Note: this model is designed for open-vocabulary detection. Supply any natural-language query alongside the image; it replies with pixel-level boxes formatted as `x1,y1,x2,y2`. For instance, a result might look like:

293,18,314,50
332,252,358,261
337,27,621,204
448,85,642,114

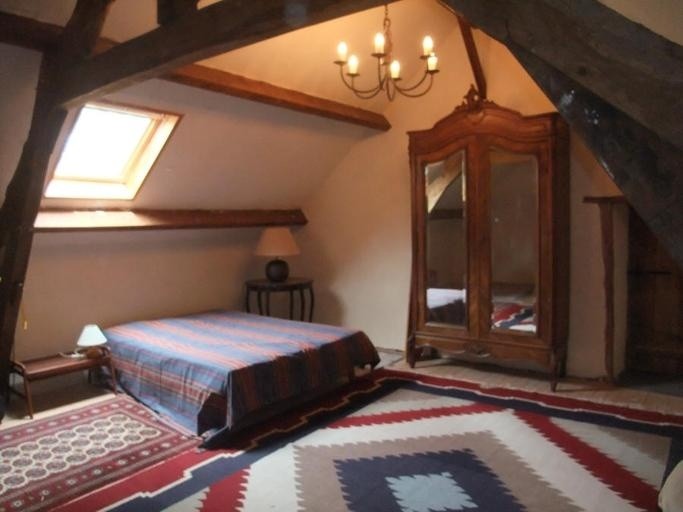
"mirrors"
488,146,539,336
421,148,470,329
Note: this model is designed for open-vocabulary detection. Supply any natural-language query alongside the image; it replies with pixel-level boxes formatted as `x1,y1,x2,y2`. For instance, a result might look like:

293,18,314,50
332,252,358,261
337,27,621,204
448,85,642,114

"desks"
243,276,315,323
3,347,120,418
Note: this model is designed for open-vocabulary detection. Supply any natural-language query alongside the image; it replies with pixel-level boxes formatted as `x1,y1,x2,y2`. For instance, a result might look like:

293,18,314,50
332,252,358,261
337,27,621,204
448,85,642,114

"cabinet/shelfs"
405,85,570,392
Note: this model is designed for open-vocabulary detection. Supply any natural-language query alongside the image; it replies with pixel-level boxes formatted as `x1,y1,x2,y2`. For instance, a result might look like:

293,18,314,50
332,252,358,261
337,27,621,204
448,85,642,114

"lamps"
254,227,302,283
333,4,441,102
76,324,105,357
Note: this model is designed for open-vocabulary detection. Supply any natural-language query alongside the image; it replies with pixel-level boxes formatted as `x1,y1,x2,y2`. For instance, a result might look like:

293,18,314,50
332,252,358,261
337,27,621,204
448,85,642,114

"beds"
102,308,367,424
